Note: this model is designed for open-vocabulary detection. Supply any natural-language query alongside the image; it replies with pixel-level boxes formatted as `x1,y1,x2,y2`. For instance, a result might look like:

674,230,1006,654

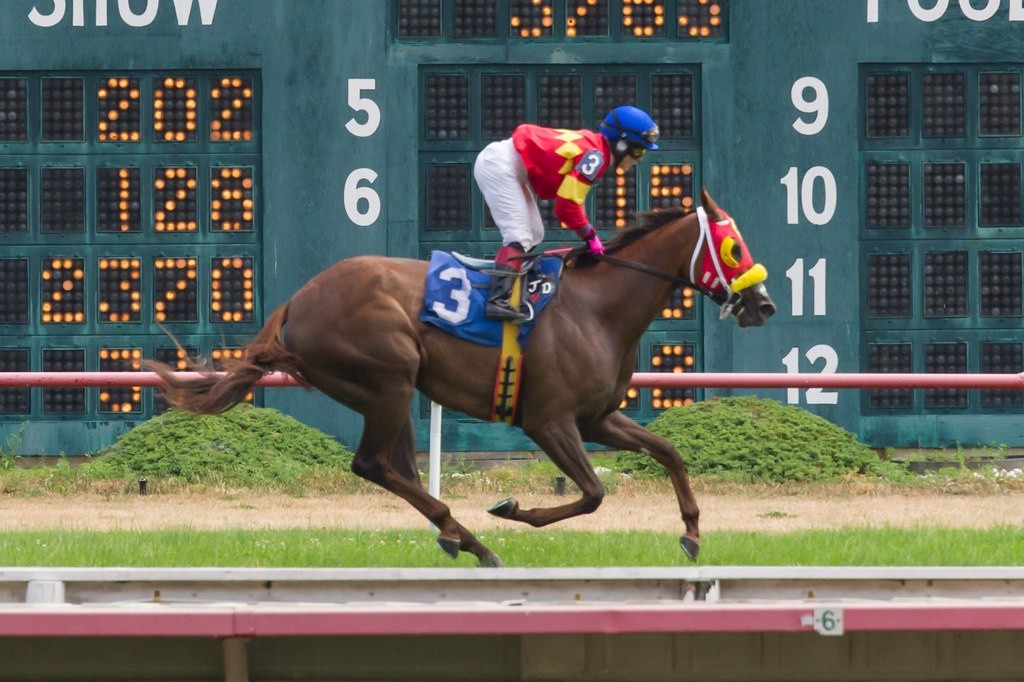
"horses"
141,185,777,568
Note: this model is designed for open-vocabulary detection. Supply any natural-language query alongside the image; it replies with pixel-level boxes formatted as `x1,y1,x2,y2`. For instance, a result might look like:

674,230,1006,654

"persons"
473,105,660,324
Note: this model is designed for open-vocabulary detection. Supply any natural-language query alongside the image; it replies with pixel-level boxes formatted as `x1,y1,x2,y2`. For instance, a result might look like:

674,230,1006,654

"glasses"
642,126,659,143
627,143,647,159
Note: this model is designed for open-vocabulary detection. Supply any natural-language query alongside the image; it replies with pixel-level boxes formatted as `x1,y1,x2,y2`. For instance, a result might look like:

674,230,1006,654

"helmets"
599,106,659,151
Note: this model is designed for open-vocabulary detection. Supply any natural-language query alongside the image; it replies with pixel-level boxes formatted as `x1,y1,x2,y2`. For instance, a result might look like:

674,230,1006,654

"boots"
483,241,536,322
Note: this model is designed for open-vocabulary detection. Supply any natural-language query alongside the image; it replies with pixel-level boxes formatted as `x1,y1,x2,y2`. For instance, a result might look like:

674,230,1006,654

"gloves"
585,228,605,256
